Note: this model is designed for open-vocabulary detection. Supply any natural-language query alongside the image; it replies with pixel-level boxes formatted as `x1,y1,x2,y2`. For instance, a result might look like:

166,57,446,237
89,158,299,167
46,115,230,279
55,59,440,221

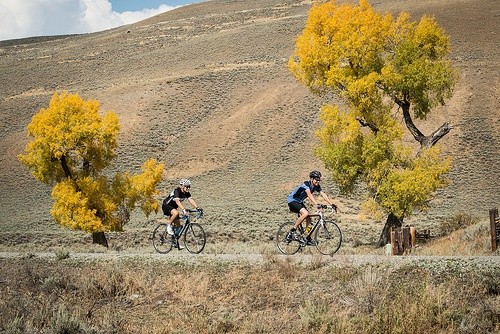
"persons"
285,170,337,246
162,179,203,249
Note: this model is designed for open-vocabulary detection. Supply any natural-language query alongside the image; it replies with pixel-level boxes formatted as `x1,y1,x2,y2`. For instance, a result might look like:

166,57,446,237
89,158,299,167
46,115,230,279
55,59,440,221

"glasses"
315,178,320,181
184,185,190,189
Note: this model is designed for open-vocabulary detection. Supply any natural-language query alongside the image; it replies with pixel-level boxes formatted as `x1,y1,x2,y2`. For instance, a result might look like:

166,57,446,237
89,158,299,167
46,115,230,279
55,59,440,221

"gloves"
331,204,337,210
317,204,324,210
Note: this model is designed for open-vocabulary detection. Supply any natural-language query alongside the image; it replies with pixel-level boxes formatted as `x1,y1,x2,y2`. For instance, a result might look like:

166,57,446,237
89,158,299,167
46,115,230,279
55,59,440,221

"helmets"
180,178,191,186
309,170,322,178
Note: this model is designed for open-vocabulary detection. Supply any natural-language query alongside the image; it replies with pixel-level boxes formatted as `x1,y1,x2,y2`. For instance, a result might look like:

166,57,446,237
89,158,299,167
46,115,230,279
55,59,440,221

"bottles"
305,223,313,234
176,226,183,235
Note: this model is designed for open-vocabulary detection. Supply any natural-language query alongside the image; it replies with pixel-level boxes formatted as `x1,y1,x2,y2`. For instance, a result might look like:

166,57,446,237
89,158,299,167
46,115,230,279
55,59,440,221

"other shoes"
179,245,186,249
290,229,299,241
166,224,175,236
309,238,319,245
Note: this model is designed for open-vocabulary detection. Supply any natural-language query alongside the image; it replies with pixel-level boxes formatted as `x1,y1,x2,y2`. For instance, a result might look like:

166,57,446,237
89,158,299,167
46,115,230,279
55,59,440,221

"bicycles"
276,204,342,255
153,207,206,254
